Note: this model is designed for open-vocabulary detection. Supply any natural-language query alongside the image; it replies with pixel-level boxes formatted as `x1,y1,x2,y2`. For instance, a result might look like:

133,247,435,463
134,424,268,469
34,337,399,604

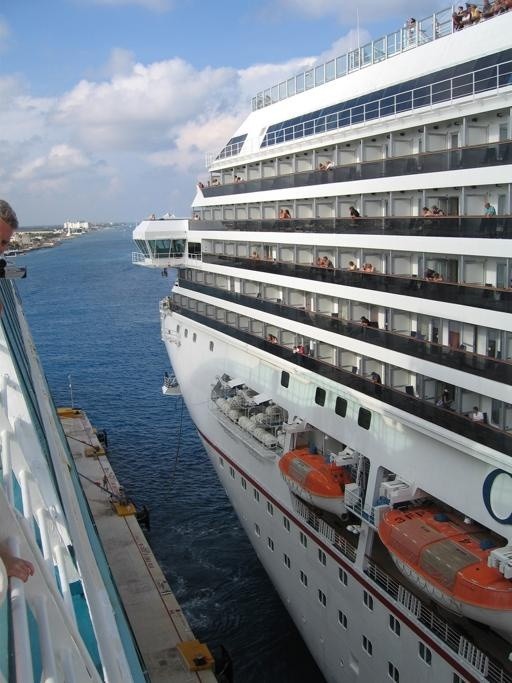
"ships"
0,236,227,681
129,0,510,683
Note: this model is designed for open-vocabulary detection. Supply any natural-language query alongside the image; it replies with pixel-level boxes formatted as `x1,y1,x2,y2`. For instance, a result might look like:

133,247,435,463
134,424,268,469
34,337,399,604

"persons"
471,405,483,422
371,370,382,394
234,174,239,182
237,175,244,181
1,199,18,257
279,208,284,217
0,538,35,581
293,345,301,354
194,214,201,220
433,206,450,232
404,16,418,47
324,156,335,181
272,335,279,344
267,333,274,342
441,388,453,407
359,315,370,327
214,178,220,185
451,0,512,31
103,474,109,488
253,250,259,259
283,209,291,218
315,160,326,183
424,271,442,281
199,181,204,187
313,253,375,272
348,204,361,226
297,345,305,355
418,205,433,226
483,201,499,236
